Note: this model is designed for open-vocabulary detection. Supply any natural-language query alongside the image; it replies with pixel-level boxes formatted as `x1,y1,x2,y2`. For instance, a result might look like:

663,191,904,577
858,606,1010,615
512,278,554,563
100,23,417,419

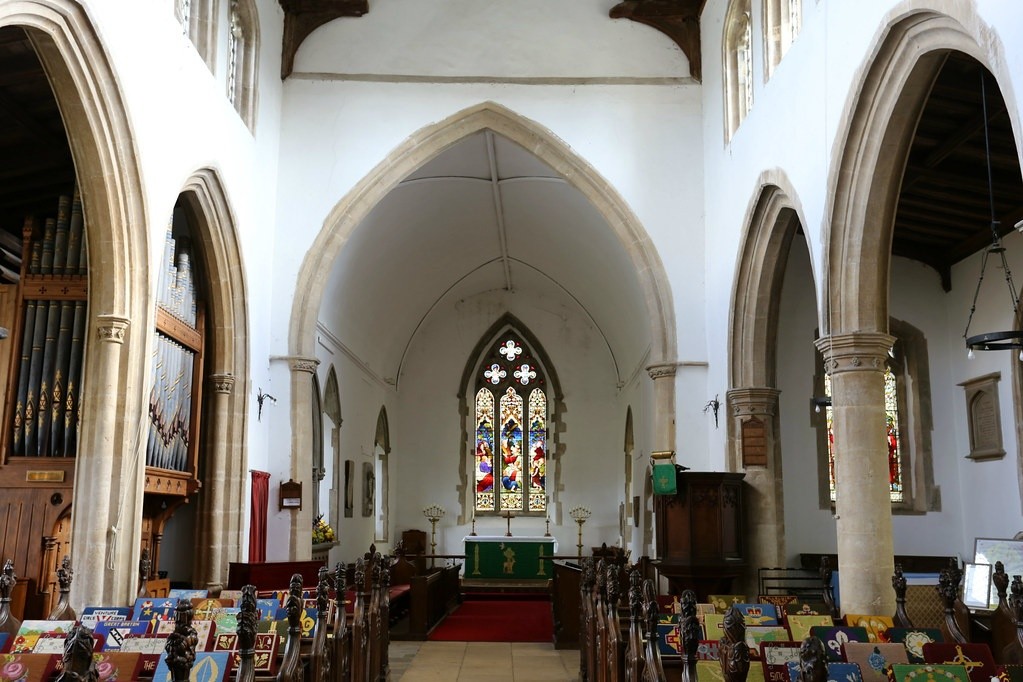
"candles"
568,504,592,514
547,504,550,521
423,504,445,513
473,505,474,519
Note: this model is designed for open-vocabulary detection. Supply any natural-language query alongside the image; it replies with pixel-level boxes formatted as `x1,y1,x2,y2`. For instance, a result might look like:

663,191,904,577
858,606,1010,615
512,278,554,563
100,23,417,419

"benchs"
578,557,1023,682
0,552,390,682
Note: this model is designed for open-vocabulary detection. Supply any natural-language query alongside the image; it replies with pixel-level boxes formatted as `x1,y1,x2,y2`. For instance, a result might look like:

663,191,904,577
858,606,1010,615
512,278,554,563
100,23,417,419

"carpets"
427,600,554,641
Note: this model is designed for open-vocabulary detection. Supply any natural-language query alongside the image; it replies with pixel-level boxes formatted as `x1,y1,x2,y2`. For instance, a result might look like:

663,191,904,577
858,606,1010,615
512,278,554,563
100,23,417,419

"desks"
464,536,555,580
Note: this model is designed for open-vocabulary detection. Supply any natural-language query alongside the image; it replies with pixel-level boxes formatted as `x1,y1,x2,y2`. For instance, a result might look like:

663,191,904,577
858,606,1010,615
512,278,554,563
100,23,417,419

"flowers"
312,514,334,545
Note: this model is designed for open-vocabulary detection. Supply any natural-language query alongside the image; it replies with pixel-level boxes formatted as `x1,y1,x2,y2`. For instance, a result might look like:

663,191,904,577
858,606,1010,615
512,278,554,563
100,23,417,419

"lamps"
961,64,1023,362
